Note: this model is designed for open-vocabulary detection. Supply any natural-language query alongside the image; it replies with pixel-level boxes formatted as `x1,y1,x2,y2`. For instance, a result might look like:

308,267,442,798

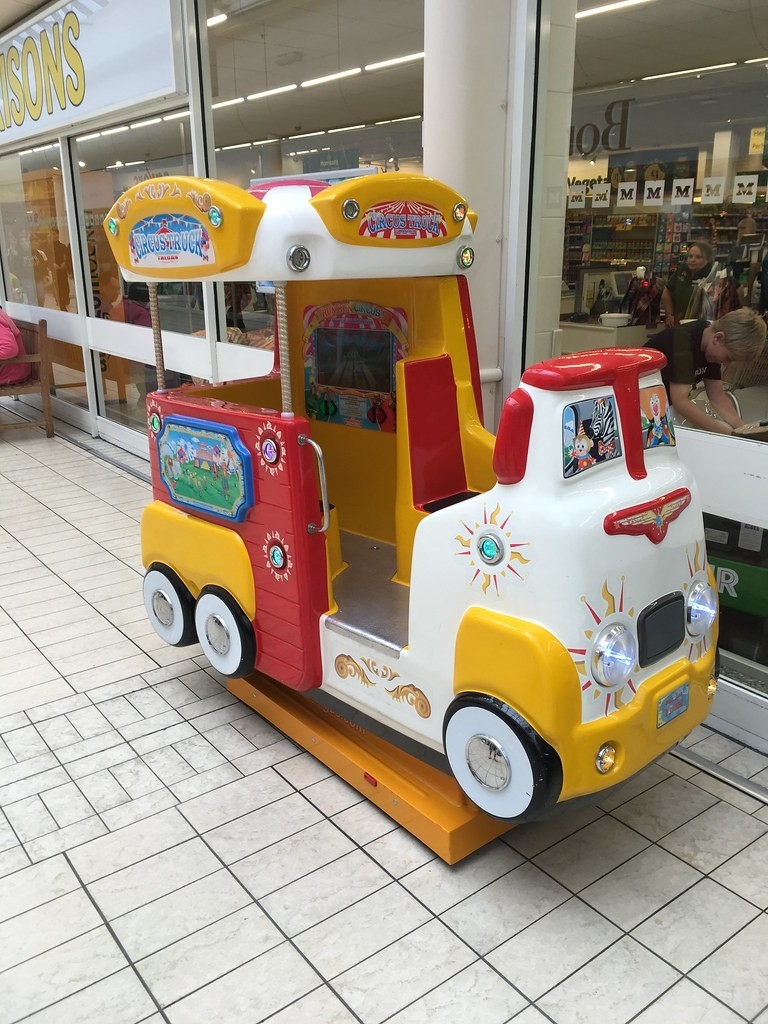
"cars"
98,163,727,823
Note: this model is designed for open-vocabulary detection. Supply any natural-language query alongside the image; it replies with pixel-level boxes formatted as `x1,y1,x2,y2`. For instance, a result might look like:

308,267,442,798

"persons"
10,236,47,307
663,239,716,330
98,274,121,318
110,283,158,408
0,307,31,385
38,229,74,312
642,308,767,436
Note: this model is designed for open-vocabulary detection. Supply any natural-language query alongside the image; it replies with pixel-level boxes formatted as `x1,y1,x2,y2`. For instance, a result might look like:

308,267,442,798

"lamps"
17,1,767,171
207,14,227,27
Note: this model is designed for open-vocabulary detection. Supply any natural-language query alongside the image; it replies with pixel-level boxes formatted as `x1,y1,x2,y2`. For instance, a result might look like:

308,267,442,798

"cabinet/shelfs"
590,214,659,270
687,212,768,268
565,219,584,286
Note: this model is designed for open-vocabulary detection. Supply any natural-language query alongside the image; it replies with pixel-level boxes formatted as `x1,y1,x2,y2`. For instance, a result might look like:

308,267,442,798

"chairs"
393,352,485,585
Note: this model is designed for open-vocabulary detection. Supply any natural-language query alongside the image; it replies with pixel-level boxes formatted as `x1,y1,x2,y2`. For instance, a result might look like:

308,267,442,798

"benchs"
0,317,53,439
